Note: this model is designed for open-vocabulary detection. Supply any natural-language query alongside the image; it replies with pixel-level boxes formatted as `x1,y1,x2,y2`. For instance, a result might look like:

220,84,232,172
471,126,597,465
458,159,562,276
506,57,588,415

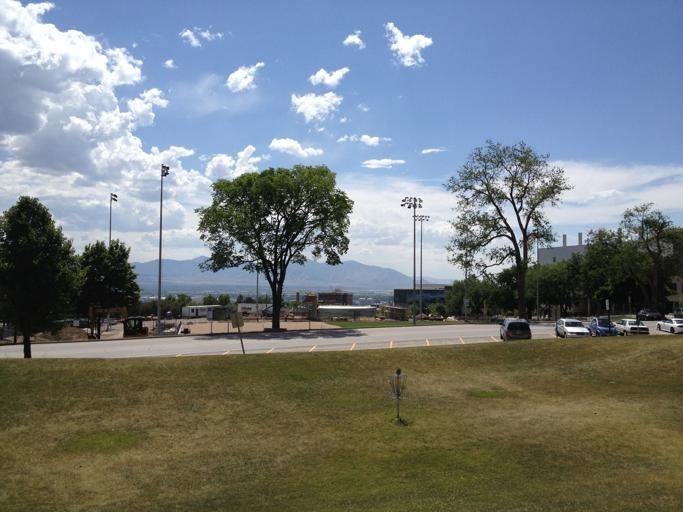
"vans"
498,318,532,342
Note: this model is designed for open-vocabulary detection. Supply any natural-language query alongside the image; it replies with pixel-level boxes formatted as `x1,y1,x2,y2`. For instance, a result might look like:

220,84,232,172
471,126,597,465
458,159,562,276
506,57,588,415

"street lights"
157,164,170,322
400,196,430,325
605,299,611,336
109,193,118,249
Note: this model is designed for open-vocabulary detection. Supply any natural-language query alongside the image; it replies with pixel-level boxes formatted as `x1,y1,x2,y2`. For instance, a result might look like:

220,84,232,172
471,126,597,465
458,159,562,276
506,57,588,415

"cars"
555,308,683,339
491,315,505,321
